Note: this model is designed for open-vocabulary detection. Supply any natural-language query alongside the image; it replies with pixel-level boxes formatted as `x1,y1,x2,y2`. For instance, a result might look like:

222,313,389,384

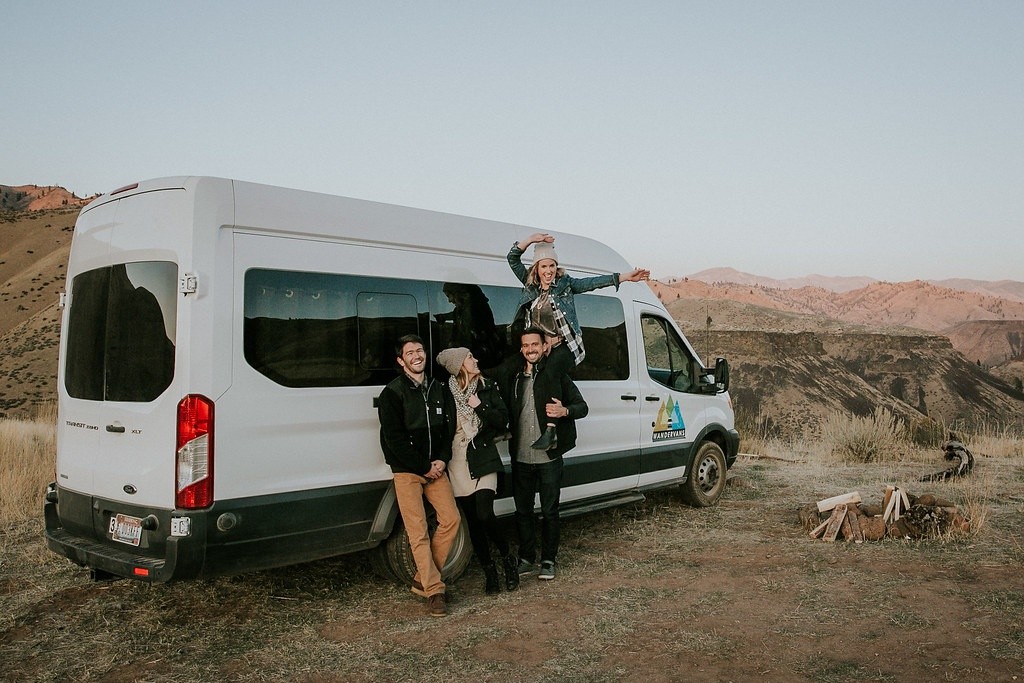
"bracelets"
566,408,568,415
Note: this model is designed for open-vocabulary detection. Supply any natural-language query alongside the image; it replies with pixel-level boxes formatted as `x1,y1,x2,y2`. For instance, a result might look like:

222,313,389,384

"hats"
436,347,470,377
531,242,558,267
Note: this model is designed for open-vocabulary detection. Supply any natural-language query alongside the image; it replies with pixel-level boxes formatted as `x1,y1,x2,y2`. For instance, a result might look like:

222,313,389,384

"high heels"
493,430,512,443
532,426,557,451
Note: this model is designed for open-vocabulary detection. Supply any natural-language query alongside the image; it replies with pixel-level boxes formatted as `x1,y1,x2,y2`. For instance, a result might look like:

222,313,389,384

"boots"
502,553,520,592
483,560,499,596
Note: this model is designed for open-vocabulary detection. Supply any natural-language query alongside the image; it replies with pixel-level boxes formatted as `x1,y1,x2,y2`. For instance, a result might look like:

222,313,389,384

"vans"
42,174,741,593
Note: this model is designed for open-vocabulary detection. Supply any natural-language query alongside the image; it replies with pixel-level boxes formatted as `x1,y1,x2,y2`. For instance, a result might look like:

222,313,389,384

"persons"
504,233,650,580
378,334,461,616
436,346,521,595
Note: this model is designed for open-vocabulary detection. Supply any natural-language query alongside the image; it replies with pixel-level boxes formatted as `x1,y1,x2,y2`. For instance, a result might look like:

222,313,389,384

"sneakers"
428,594,446,617
538,561,555,579
504,559,534,577
410,580,429,599
